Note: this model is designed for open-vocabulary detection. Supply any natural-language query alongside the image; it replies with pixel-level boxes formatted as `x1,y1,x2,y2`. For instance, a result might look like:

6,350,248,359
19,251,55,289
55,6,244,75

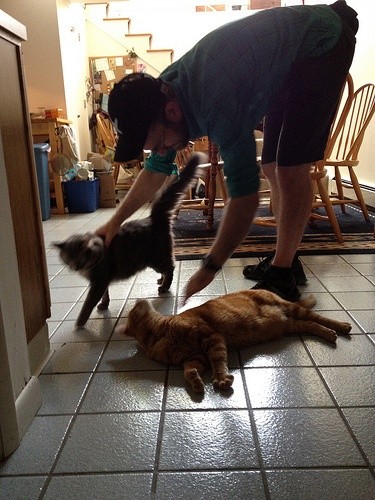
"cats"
51,152,209,329
114,290,353,393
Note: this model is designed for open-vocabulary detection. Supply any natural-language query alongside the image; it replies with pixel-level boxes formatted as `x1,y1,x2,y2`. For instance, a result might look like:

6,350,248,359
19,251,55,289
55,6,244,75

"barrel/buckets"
33,143,50,221
62,177,98,213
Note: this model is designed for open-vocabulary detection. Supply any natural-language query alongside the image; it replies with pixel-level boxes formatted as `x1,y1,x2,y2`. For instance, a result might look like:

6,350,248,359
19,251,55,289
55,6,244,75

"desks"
31,117,73,215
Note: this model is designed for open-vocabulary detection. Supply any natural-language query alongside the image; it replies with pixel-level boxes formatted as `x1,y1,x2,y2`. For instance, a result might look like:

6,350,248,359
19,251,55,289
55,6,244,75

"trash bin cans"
33,143,51,221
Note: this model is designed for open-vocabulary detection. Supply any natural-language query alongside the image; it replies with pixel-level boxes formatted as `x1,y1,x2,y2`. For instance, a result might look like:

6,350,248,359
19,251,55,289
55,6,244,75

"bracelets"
200,253,222,273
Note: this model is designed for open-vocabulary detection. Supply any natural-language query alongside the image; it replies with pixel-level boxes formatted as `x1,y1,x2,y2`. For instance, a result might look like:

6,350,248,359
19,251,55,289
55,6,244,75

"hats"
108,72,159,162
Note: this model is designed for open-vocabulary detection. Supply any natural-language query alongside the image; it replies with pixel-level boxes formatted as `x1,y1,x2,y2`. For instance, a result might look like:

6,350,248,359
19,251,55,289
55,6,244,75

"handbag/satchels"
329,0,359,35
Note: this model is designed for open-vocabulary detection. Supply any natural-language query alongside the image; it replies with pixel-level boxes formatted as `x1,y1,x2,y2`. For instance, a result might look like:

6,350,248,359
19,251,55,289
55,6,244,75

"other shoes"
249,274,302,302
243,251,308,284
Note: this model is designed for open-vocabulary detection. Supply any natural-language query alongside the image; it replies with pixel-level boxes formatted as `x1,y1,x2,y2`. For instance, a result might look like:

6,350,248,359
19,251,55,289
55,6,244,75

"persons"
95,0,359,308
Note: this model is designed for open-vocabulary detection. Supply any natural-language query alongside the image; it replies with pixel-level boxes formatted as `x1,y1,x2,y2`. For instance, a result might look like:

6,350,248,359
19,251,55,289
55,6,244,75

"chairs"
173,141,229,220
300,71,345,243
310,82,375,222
96,114,143,194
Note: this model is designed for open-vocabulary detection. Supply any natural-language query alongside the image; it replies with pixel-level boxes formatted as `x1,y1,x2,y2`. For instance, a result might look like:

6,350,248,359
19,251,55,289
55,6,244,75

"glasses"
151,126,165,155
